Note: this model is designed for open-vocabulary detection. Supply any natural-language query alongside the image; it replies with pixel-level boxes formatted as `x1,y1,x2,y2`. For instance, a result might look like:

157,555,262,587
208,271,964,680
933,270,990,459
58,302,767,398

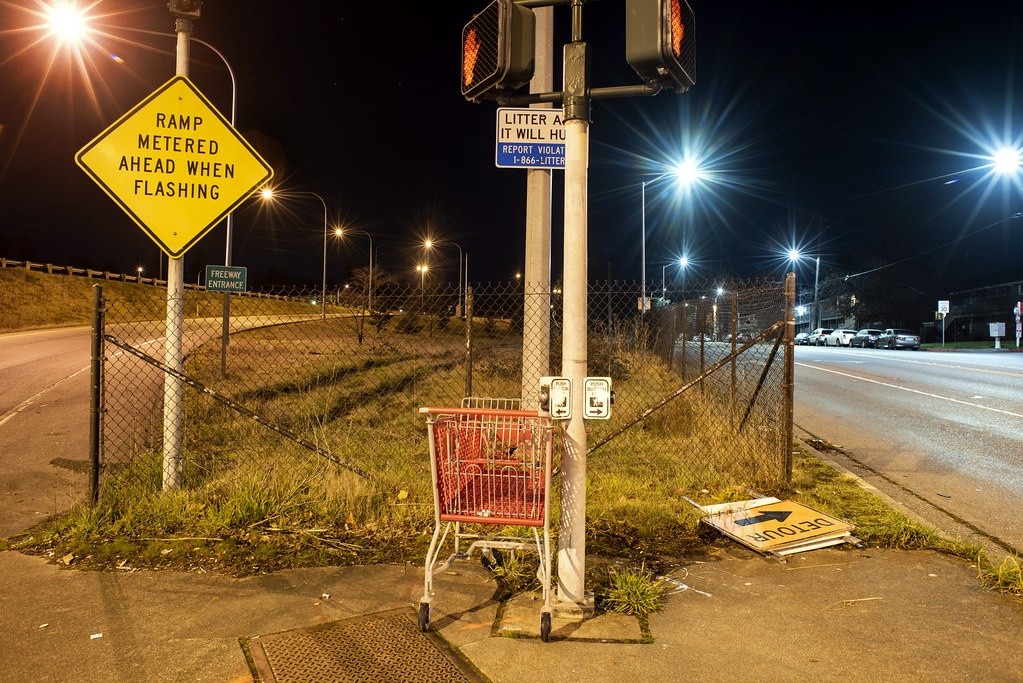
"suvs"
807,328,834,346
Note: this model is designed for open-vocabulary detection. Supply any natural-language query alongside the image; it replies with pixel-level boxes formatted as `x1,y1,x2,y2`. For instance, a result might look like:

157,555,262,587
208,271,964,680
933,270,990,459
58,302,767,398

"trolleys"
417,396,556,642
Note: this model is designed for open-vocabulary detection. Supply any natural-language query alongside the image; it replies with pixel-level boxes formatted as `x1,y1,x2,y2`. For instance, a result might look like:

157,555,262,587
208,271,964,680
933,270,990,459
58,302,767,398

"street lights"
663,258,688,304
642,165,694,313
426,241,462,314
338,228,372,313
58,8,237,372
418,264,429,312
267,190,325,320
792,252,819,330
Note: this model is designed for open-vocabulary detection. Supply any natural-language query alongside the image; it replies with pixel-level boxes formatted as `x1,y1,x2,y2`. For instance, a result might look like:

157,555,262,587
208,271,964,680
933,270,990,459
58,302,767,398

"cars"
795,332,809,345
736,333,753,343
849,329,882,349
824,329,858,347
874,329,921,350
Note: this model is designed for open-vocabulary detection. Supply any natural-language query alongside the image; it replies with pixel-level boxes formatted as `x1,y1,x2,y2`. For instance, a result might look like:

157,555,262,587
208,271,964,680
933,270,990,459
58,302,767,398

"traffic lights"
625,0,697,96
461,0,535,99
